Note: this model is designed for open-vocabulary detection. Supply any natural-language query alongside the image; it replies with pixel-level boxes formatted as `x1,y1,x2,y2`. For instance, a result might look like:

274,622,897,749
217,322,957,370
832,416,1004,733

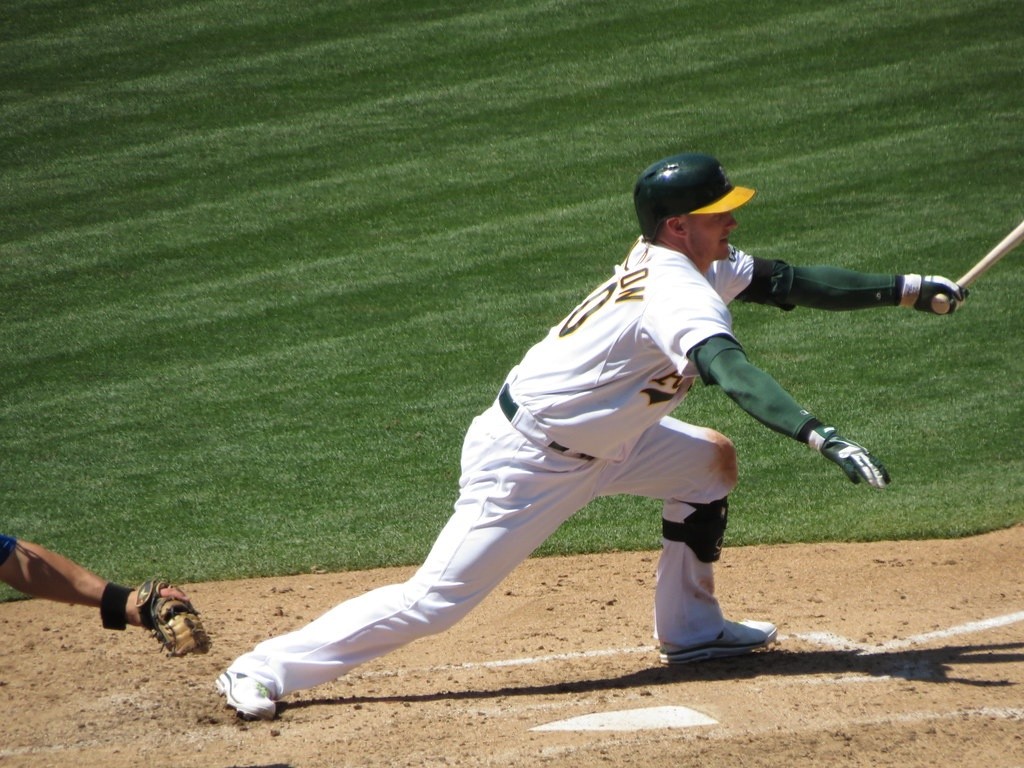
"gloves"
899,271,971,315
809,425,891,489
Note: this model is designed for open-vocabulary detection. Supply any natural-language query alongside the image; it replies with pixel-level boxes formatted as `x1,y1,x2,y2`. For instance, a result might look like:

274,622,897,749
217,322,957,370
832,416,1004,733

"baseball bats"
932,220,1024,314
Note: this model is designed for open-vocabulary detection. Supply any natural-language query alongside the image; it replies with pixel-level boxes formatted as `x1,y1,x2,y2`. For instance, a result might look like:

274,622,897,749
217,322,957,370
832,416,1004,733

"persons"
214,154,970,720
0,535,213,658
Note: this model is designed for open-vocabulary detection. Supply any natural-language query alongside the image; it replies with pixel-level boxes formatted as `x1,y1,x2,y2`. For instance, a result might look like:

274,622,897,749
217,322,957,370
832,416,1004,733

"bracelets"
99,581,134,633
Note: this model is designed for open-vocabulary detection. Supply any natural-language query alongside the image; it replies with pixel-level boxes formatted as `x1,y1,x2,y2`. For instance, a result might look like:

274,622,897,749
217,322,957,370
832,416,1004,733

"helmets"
634,154,756,244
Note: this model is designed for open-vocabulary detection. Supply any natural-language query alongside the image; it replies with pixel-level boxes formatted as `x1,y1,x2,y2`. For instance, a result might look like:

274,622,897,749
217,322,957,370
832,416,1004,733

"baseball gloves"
137,577,210,659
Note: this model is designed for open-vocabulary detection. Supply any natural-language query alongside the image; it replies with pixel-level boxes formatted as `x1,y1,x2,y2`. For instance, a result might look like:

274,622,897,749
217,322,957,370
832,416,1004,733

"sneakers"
659,621,777,664
216,656,277,719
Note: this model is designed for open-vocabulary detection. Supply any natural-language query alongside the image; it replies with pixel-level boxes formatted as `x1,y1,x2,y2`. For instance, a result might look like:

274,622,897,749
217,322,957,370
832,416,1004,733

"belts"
499,387,596,460
